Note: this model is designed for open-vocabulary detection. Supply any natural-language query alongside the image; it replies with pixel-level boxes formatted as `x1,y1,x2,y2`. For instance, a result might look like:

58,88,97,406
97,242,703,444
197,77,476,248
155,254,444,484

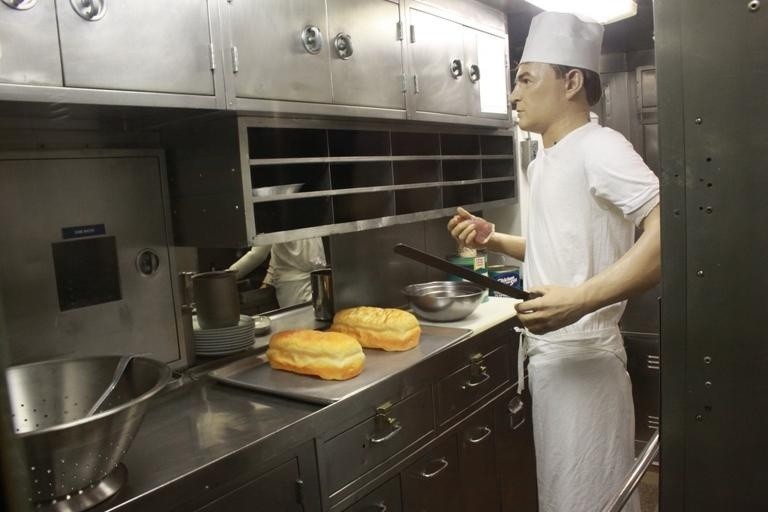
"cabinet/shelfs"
227,0,409,122
412,1,519,128
0,0,225,107
165,115,521,248
218,315,537,511
168,381,322,510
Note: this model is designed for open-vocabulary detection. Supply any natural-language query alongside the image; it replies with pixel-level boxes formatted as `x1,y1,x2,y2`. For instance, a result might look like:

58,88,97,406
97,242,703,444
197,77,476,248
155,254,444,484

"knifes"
393,242,540,301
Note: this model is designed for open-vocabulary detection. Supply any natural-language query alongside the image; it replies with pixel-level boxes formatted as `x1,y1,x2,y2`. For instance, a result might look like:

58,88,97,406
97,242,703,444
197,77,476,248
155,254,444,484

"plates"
250,182,303,197
190,315,256,356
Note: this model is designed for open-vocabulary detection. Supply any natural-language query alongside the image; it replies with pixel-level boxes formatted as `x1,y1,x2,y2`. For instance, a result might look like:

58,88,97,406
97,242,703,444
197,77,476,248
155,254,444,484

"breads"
266,329,366,380
330,306,421,352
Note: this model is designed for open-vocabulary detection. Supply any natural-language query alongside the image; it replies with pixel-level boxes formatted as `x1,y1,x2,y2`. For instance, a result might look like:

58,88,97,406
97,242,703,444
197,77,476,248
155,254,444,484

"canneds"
488,265,521,298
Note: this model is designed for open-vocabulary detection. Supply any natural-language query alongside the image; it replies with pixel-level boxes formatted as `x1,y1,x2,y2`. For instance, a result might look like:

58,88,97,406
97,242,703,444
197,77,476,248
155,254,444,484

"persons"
446,10,662,512
224,236,327,308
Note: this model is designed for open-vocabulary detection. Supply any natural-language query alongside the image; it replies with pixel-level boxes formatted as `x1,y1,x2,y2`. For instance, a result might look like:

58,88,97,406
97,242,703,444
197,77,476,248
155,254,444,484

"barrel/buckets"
448,253,488,302
487,263,520,295
310,270,331,321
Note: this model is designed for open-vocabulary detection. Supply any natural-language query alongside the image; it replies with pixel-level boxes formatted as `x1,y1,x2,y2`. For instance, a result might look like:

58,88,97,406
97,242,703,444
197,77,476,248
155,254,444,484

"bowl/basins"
1,356,173,508
252,316,271,334
402,280,486,321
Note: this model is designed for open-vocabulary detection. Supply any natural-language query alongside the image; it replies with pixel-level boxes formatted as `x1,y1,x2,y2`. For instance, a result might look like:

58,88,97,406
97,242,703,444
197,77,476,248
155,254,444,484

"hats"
519,11,606,75
